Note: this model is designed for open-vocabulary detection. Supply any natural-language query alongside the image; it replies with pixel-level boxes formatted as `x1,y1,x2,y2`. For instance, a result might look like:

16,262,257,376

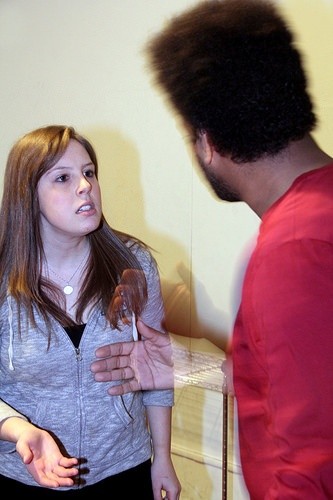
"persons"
0,125,182,500
91,1,333,500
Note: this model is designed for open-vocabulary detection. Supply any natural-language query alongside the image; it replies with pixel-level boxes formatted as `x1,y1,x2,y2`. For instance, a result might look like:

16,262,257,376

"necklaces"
49,250,89,295
